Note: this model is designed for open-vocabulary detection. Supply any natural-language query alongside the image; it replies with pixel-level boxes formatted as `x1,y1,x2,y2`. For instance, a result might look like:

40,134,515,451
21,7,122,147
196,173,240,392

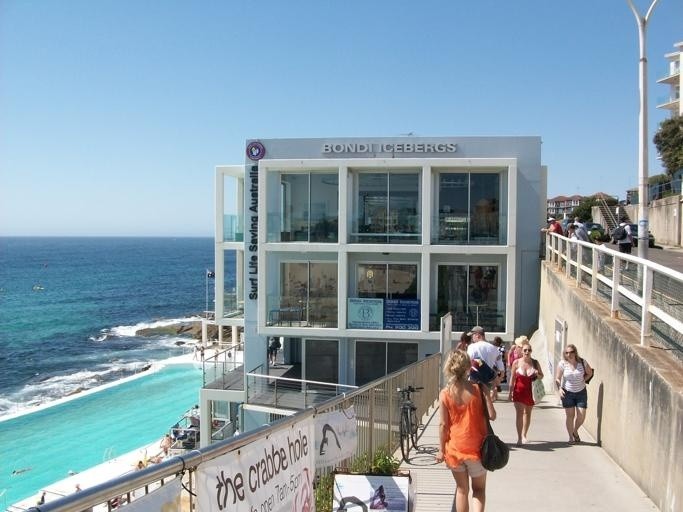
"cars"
610,223,655,248
562,217,605,239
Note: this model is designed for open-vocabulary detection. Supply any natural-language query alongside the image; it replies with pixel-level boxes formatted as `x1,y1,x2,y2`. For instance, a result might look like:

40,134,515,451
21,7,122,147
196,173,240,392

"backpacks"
613,224,629,240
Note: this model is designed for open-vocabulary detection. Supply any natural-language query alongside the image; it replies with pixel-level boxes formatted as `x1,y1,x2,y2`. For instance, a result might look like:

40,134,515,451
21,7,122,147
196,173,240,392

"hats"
514,336,529,348
619,216,629,222
547,218,555,223
465,326,484,336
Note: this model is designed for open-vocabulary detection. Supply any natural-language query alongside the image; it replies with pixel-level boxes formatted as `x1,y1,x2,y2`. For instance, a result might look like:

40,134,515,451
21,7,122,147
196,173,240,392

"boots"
568,432,580,444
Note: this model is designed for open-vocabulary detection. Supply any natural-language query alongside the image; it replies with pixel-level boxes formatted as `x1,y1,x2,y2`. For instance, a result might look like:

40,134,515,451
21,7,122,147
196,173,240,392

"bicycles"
397,386,424,462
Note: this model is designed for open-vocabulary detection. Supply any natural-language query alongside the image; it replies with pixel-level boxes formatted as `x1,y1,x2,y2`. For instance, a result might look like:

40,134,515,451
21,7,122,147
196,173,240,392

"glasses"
523,348,531,352
566,352,574,354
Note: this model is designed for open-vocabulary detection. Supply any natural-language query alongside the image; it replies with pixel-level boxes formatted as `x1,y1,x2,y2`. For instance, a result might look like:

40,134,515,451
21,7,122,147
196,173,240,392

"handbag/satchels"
479,433,508,472
468,359,497,384
581,357,594,384
531,378,545,404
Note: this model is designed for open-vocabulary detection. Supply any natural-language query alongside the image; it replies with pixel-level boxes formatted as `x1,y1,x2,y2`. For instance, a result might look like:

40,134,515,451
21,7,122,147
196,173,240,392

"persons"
489,337,508,393
480,276,489,300
433,348,498,512
505,335,528,364
572,217,585,230
191,345,198,360
616,216,635,271
199,346,204,360
539,217,563,235
565,222,590,242
212,348,219,364
226,350,232,361
268,337,276,365
134,460,145,471
160,433,171,454
504,339,543,447
463,325,501,394
627,200,630,205
553,343,593,445
455,332,471,353
